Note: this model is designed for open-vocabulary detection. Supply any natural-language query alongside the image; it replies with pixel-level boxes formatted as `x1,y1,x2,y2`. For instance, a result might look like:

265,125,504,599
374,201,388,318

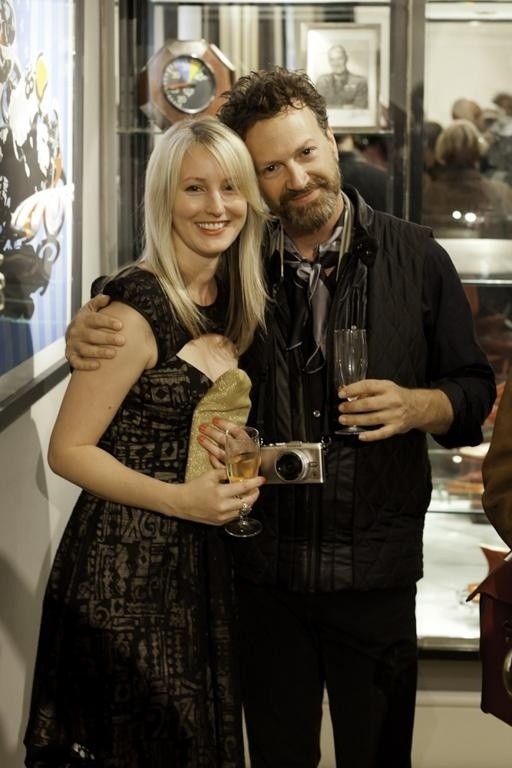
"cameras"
257,442,324,485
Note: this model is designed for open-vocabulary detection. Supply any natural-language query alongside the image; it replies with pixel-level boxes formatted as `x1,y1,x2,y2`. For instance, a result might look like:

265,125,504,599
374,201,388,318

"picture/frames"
299,22,381,135
0,0,85,434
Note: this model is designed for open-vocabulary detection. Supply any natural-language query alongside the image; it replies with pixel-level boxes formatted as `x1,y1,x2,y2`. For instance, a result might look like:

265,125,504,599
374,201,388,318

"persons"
313,44,369,109
306,87,512,325
21,112,279,768
59,64,501,767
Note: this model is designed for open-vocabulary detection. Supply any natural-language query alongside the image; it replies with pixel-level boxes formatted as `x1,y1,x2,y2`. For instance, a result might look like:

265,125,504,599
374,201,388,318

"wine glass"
332,327,372,436
222,427,264,538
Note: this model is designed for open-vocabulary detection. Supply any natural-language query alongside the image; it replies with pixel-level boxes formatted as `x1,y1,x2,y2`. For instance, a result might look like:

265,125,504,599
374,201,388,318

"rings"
239,502,252,517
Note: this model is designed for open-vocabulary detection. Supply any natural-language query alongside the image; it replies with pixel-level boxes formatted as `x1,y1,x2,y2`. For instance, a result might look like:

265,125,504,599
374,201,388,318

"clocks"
134,41,237,135
